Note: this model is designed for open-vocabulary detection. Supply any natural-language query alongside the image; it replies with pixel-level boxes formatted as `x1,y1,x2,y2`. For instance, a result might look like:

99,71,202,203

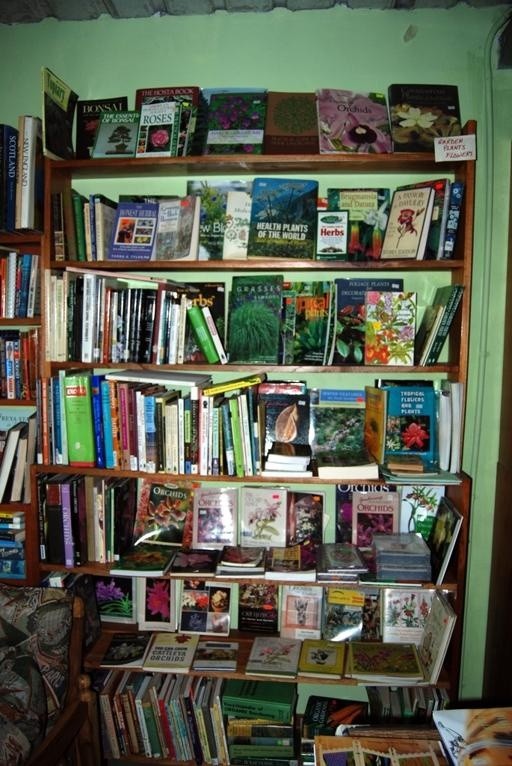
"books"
432,707,512,766
0,63,462,766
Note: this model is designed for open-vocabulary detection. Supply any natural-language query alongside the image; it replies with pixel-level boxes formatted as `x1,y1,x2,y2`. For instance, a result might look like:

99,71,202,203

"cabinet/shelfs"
0,121,478,766
